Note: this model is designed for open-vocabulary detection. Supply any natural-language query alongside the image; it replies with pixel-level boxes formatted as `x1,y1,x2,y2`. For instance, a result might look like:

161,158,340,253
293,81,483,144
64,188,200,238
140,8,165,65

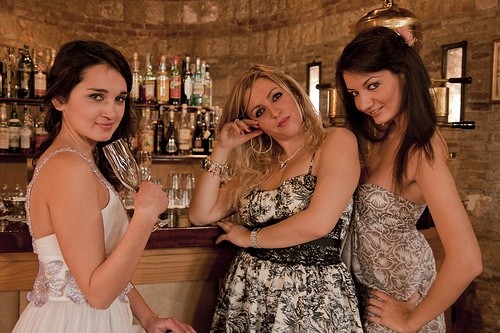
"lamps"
356,0,419,28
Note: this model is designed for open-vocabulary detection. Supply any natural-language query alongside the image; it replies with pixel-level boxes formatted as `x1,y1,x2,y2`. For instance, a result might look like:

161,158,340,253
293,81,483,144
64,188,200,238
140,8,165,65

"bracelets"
200,157,228,176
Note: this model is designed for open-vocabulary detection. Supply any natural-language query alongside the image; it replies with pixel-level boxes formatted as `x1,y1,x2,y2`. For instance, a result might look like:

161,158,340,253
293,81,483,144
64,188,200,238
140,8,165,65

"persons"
335,25,483,333
187,64,363,333
9,39,197,333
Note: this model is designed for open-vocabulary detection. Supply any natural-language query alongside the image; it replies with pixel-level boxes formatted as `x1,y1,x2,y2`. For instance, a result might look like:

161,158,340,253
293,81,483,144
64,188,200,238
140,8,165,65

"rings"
235,119,239,122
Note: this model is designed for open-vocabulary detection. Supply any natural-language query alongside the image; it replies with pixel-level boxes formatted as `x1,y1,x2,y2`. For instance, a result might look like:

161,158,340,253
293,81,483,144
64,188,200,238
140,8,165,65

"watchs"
250,228,260,249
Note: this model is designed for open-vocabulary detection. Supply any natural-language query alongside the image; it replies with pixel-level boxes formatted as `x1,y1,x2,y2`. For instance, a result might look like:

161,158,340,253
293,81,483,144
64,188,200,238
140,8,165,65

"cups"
150,173,197,228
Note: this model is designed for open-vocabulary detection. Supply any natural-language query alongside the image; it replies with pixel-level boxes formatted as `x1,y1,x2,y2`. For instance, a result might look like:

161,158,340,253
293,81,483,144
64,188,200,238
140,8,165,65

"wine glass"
102,137,171,233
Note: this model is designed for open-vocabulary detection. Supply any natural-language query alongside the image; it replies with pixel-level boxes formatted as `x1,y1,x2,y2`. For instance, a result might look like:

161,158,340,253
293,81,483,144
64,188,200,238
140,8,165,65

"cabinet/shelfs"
0,97,212,163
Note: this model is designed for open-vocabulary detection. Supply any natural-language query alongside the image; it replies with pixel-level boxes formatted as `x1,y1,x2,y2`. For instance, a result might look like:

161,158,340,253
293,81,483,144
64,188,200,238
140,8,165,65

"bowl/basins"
3,197,26,213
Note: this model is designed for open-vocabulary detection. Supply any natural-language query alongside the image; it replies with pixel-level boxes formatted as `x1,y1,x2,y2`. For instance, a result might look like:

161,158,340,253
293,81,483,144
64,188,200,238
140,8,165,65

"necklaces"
278,145,303,171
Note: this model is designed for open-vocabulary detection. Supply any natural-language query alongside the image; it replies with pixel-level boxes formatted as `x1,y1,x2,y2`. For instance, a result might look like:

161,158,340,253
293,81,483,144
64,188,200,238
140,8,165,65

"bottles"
0,44,223,156
